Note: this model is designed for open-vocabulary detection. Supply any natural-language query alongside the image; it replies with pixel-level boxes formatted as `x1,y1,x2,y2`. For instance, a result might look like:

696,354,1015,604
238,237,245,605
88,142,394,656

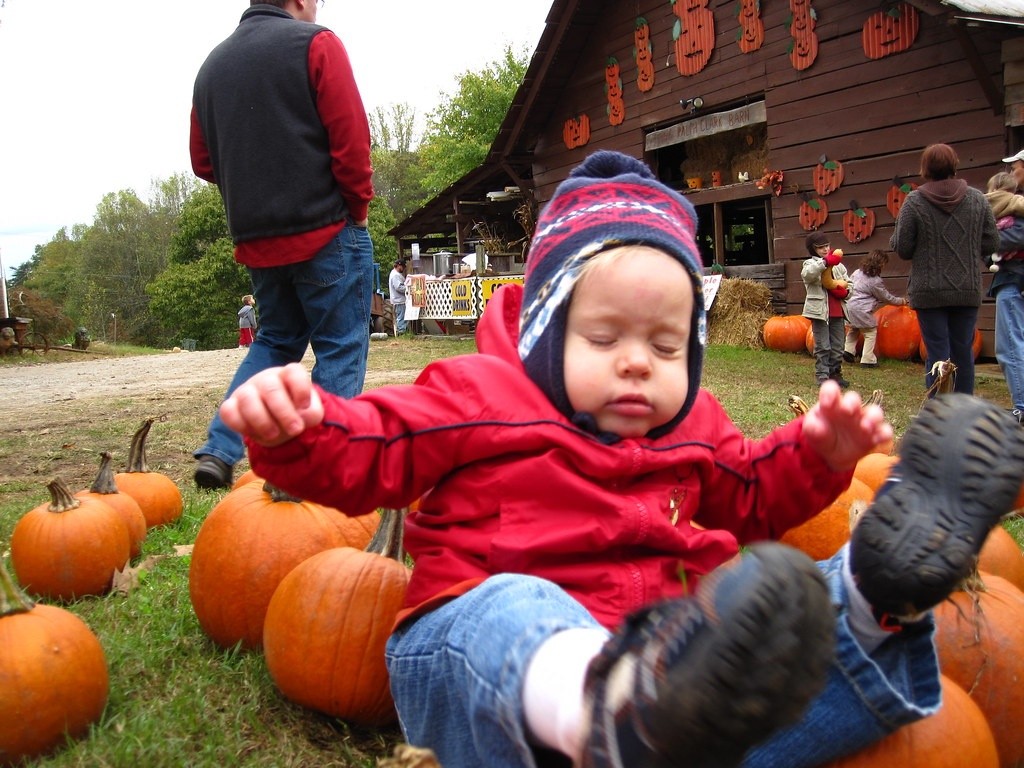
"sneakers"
1008,407,1024,424
194,452,233,493
851,391,1024,635
580,543,834,768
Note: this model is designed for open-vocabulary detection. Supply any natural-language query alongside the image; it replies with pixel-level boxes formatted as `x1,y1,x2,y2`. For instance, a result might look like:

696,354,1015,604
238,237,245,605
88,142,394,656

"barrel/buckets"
432,249,455,276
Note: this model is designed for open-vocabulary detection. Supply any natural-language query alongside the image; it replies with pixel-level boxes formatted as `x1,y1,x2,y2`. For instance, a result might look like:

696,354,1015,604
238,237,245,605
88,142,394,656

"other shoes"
863,363,880,368
843,352,854,363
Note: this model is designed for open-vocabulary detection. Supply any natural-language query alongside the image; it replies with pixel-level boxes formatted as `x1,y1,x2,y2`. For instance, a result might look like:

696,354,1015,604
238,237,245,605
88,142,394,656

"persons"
188,1,374,497
237,295,257,349
388,259,409,335
800,231,853,388
403,255,423,336
219,151,1024,768
980,149,1024,426
982,172,1024,274
889,142,1004,400
844,248,911,369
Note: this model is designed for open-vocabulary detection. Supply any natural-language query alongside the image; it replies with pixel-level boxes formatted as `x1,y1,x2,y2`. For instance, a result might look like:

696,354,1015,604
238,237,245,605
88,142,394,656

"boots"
830,369,849,387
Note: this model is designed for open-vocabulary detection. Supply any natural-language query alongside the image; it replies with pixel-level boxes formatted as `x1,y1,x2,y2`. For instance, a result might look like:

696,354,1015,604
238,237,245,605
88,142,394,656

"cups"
453,263,470,274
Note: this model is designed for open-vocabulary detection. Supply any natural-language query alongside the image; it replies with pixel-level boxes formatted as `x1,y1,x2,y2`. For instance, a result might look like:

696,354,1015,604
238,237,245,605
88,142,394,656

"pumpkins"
773,360,1024,768
822,249,849,290
764,298,982,365
0,415,415,767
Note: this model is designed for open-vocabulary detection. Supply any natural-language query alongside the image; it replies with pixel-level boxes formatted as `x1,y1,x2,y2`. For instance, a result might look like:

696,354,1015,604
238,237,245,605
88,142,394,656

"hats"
1002,150,1024,163
517,151,706,444
806,231,830,257
394,259,406,265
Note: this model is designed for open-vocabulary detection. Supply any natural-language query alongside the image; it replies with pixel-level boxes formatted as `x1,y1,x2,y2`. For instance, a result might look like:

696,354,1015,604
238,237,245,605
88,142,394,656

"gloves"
830,284,848,300
823,249,842,266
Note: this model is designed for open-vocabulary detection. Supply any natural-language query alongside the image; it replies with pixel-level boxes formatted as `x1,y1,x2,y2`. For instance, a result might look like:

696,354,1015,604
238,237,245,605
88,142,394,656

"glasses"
317,0,325,8
